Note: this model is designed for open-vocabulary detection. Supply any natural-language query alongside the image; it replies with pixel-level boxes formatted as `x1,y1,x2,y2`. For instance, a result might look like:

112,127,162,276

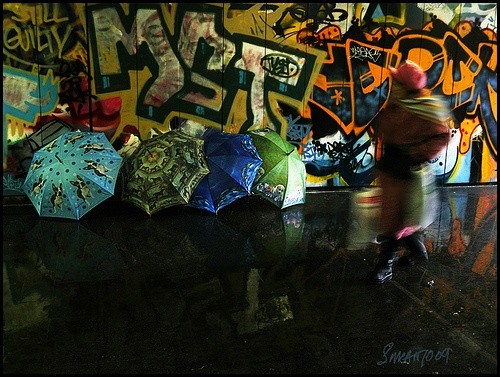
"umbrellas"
250,129,306,210
20,129,123,222
120,128,211,217
184,130,263,212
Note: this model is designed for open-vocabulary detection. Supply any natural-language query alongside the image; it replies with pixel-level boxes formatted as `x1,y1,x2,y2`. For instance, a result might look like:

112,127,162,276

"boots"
366,251,394,285
397,250,428,266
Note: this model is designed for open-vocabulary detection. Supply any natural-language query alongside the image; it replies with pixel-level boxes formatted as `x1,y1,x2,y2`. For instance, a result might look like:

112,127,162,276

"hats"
388,59,426,89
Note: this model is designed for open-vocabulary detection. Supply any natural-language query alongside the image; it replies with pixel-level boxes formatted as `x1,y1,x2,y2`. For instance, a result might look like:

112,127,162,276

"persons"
373,62,451,281
